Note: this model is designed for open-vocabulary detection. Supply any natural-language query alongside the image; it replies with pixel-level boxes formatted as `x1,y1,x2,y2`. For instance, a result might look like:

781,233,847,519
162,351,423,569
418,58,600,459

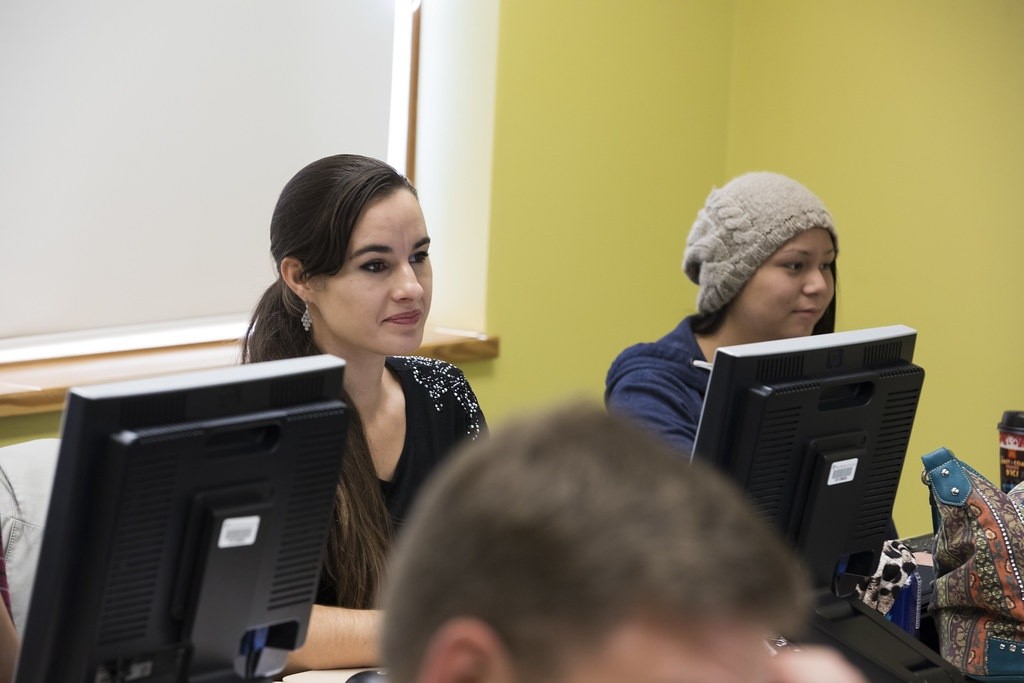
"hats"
684,171,834,314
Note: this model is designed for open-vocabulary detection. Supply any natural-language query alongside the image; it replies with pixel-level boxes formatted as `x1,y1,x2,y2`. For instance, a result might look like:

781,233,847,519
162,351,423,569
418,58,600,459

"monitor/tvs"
684,326,969,683
12,354,353,683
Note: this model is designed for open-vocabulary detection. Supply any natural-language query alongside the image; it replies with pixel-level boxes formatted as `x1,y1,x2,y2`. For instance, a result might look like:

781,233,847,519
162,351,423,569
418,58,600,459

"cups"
997,410,1024,492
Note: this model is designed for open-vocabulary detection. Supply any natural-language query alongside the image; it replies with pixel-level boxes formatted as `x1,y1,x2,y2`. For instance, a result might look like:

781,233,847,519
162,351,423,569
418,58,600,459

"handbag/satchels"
922,446,1023,682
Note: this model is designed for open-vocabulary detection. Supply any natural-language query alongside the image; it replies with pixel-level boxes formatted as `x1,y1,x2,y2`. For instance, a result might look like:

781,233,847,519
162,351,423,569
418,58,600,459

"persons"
244,153,491,670
603,171,839,461
380,400,811,683
0,438,62,683
